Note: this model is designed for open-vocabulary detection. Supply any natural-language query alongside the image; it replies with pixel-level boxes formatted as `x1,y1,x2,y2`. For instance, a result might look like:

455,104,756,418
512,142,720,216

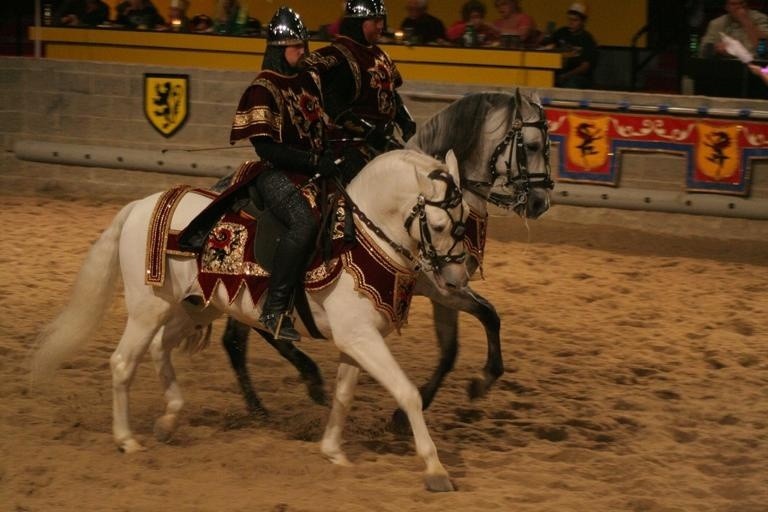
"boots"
260,238,308,340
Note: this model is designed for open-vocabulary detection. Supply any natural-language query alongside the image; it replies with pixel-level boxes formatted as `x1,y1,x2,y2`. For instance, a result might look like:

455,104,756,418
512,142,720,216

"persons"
697,0,768,60
229,8,367,346
365,0,540,53
305,0,417,182
540,0,595,89
64,0,262,37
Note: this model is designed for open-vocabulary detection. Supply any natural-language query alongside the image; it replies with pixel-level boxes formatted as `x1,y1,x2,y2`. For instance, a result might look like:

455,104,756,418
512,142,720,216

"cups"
37,1,573,54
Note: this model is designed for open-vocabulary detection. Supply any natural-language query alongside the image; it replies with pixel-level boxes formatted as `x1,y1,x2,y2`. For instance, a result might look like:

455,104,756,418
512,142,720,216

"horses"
208,86,550,425
23,149,469,491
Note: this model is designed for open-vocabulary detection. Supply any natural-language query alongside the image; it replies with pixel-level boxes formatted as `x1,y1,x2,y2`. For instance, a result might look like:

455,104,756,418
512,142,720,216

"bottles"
688,15,703,58
756,24,768,60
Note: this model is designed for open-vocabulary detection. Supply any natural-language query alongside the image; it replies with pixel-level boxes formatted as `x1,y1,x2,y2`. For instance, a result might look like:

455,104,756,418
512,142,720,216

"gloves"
383,135,405,152
310,150,340,177
400,115,415,135
339,147,353,162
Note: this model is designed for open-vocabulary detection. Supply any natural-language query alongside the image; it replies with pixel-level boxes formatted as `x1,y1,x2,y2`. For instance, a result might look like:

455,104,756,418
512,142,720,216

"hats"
568,3,587,17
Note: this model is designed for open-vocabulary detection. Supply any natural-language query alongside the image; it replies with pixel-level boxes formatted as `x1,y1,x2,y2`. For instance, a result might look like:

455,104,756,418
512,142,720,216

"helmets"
268,6,310,47
343,0,387,17
